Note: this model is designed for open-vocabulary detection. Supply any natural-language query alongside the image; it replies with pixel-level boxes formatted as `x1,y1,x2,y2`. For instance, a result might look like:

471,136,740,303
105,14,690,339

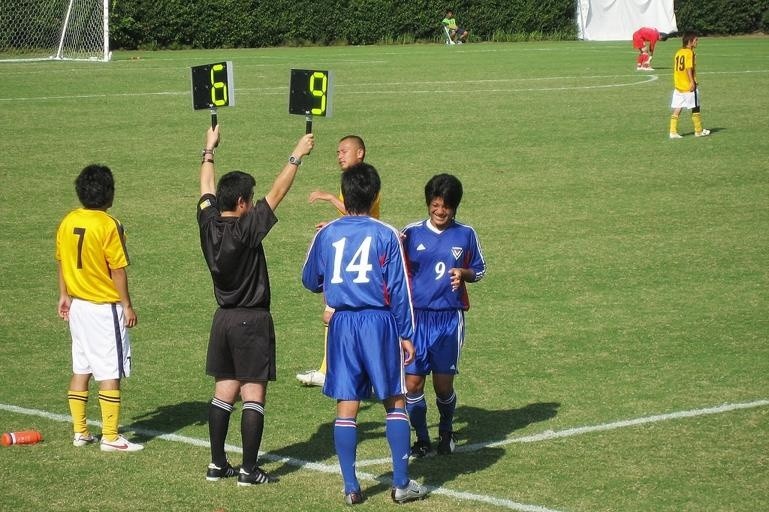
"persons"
55,163,146,453
632,27,668,72
300,162,429,504
196,123,316,488
394,172,488,460
441,10,468,45
294,134,387,388
669,30,711,139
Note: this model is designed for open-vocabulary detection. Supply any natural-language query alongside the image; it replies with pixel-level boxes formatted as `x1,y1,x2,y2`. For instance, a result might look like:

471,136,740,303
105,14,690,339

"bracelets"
201,148,215,156
201,158,215,164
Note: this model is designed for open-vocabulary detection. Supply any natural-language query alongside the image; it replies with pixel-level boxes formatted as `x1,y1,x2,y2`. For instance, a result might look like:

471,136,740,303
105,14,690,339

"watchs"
288,155,301,166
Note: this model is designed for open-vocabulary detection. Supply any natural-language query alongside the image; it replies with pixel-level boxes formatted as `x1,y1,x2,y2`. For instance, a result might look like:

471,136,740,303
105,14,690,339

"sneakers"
297,370,325,386
238,466,273,486
412,431,455,457
73,432,144,451
206,462,236,481
346,491,362,504
669,129,710,138
392,480,429,503
